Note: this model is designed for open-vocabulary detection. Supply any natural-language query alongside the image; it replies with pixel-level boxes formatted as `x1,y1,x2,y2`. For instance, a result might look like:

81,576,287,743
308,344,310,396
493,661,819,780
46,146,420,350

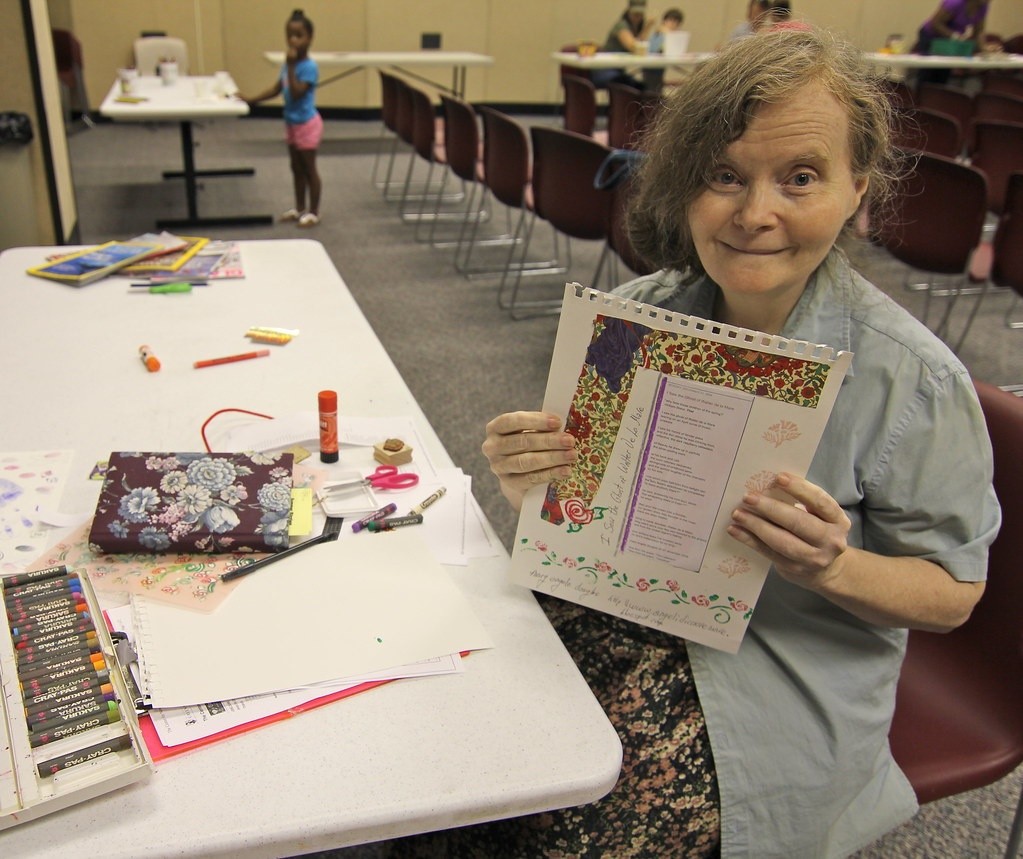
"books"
25,227,245,288
88,344,294,555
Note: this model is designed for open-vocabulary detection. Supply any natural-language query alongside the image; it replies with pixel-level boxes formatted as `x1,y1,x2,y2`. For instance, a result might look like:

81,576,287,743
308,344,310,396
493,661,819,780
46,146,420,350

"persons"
585,0,989,93
234,9,325,227
457,29,1005,859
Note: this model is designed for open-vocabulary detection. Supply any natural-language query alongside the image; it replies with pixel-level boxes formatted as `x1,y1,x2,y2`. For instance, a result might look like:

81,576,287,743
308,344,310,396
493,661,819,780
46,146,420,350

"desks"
100,71,272,229
553,51,1023,96
264,51,493,102
0,239,622,859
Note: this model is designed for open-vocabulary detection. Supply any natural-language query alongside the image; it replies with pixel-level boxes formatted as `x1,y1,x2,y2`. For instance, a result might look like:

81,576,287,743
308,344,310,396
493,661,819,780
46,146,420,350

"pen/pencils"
196,350,268,369
222,532,333,581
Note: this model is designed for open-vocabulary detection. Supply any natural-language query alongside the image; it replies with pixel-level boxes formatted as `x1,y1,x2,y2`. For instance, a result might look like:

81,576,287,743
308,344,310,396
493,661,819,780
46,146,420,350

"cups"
118,68,140,97
635,41,649,56
158,62,178,84
663,30,690,57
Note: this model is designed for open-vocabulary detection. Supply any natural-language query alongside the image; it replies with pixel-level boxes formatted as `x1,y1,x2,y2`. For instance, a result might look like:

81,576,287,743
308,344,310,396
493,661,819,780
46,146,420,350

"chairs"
135,35,205,129
372,66,1023,359
887,380,1023,859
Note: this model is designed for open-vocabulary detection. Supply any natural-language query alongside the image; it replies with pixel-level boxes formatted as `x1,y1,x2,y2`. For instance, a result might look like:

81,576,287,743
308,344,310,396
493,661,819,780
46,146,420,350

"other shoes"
300,212,321,226
281,209,304,222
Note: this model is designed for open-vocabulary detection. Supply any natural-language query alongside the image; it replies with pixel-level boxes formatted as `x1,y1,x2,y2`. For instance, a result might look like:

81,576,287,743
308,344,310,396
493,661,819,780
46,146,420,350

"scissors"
324,463,418,493
